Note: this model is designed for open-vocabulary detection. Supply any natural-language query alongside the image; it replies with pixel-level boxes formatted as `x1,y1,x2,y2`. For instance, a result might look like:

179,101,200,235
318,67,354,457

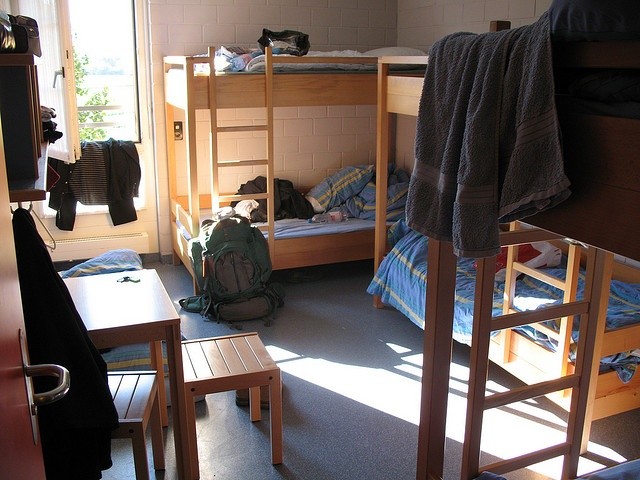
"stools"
108,370,166,479
181,331,284,479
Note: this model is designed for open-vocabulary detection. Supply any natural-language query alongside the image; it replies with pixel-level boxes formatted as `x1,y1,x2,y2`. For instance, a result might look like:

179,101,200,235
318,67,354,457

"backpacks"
179,218,284,330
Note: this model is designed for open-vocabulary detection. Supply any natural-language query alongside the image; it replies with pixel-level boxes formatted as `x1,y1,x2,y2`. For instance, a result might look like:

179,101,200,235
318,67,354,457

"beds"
414,20,640,479
372,55,639,454
163,45,432,298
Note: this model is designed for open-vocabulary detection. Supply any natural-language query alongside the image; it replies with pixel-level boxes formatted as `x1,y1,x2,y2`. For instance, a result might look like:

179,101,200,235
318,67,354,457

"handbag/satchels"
472,241,562,281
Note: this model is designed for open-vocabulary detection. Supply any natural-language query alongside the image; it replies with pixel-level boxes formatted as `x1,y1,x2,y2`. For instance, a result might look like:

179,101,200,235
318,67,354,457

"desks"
56,268,182,473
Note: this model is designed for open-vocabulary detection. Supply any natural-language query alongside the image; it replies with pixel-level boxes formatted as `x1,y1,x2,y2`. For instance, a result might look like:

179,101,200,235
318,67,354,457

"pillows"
365,46,428,69
305,164,375,213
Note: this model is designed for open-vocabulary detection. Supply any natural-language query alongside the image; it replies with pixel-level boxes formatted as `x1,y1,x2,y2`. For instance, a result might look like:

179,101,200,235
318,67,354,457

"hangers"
16,203,57,254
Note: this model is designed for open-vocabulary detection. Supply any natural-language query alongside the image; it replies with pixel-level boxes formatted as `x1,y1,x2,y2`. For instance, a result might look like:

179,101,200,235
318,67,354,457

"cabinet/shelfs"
0,52,49,202
